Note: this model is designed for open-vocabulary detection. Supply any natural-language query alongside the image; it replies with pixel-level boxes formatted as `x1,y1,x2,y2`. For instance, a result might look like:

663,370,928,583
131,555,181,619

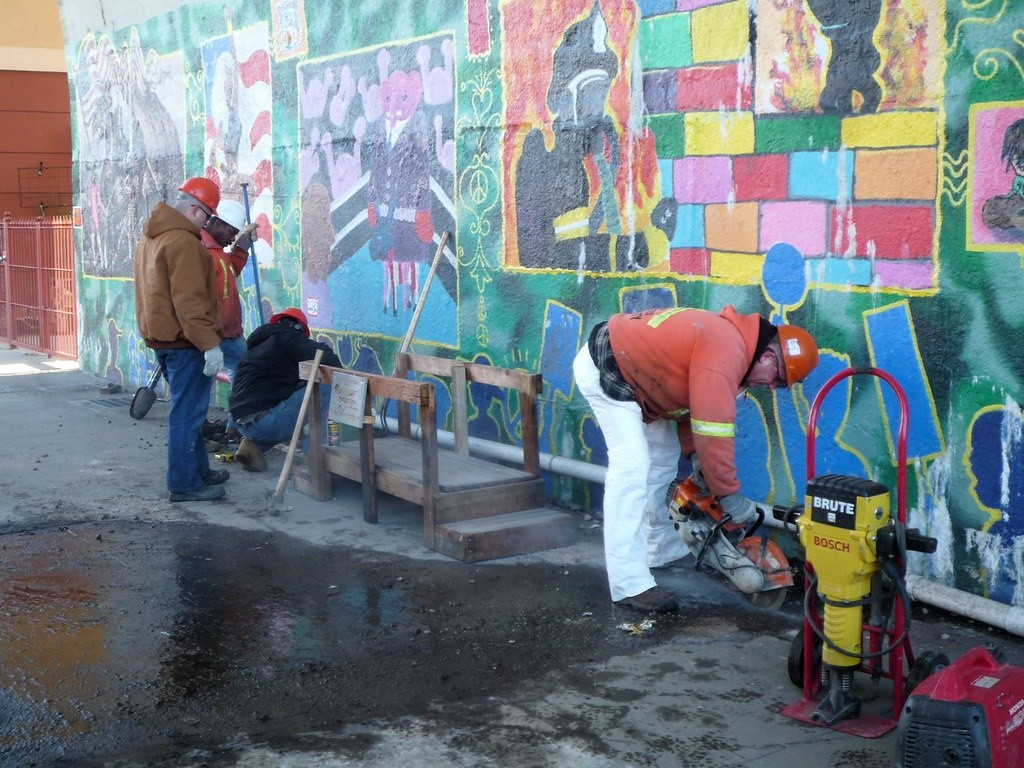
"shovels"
129,362,163,419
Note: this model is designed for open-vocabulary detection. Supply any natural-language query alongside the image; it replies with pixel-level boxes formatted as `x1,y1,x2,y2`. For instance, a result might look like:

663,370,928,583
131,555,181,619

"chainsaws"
666,481,794,615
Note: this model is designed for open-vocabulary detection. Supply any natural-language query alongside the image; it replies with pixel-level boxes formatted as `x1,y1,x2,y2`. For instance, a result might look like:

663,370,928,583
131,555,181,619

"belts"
236,410,267,428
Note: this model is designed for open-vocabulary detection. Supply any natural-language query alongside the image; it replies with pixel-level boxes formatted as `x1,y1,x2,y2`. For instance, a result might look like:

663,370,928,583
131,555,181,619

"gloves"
720,492,756,524
235,223,259,252
203,346,223,376
691,452,706,489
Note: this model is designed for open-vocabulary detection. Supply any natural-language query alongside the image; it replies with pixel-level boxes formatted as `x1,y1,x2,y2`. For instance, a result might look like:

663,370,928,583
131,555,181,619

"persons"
232,308,342,473
572,304,818,611
135,178,230,501
200,197,260,452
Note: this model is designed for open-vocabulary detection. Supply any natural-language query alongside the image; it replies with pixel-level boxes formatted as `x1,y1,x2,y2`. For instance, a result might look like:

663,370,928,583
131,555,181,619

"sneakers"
650,552,697,569
235,439,266,472
615,586,679,612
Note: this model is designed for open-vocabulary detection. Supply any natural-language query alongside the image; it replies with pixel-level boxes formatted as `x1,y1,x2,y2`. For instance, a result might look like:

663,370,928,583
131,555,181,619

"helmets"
178,178,219,216
271,308,310,338
778,325,818,390
210,200,246,231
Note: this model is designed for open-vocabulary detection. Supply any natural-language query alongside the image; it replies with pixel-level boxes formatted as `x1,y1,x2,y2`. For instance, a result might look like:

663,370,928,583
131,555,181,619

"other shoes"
202,469,229,484
169,485,226,501
204,439,219,453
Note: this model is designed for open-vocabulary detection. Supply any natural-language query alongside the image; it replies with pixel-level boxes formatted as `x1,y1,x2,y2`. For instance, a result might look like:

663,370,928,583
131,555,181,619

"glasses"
767,347,786,387
191,205,211,220
